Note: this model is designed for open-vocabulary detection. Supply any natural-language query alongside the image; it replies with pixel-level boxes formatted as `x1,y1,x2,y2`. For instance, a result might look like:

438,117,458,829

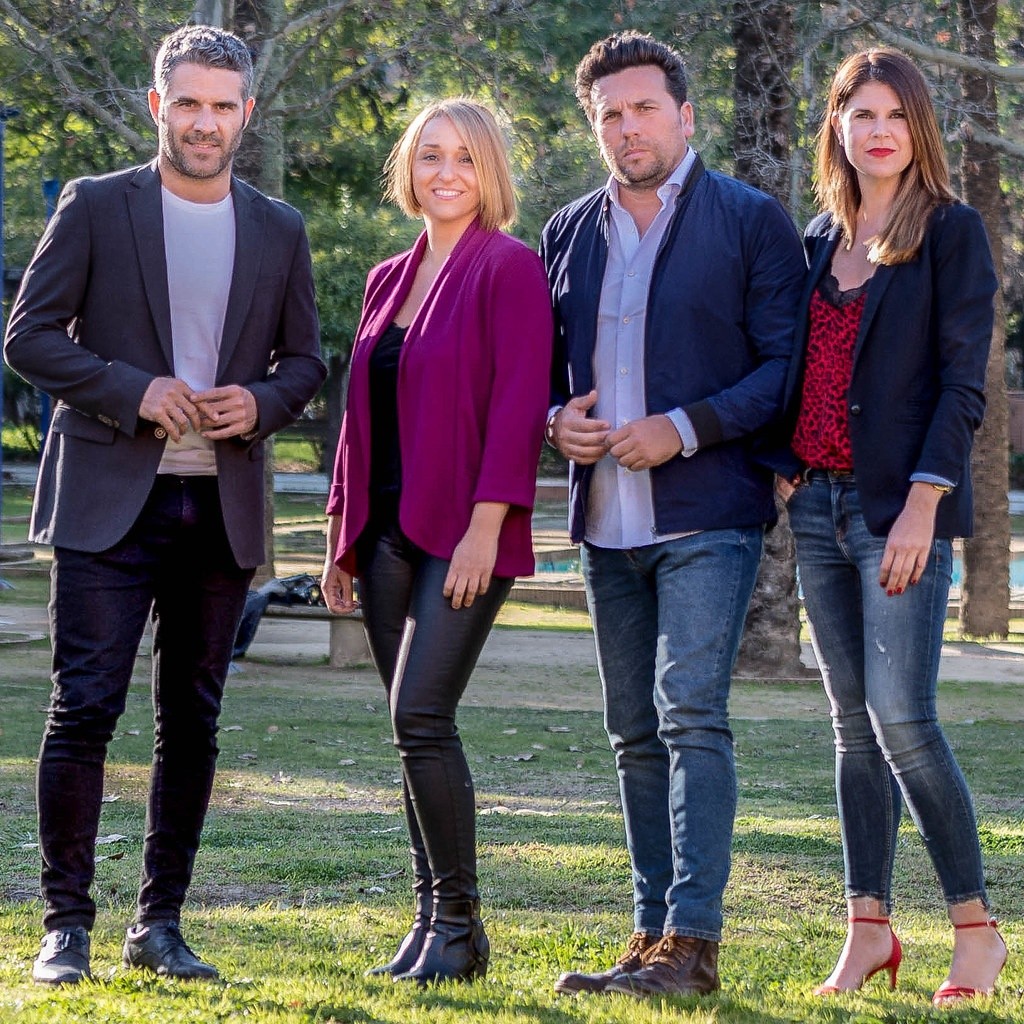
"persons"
320,99,557,983
536,27,807,1000
4,26,329,982
770,47,1012,1010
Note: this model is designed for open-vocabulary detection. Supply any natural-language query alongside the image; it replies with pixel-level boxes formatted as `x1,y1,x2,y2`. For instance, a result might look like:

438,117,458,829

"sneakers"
604,932,722,1005
552,932,662,995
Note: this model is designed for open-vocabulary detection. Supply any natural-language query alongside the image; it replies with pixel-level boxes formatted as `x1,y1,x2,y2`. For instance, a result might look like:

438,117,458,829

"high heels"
812,918,902,997
930,917,1008,1010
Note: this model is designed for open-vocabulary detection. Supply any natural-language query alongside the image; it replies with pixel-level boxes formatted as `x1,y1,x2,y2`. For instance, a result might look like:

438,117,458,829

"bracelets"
933,484,950,492
546,409,561,440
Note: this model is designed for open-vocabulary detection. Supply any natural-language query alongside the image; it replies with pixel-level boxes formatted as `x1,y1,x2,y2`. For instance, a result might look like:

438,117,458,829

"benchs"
237,601,374,670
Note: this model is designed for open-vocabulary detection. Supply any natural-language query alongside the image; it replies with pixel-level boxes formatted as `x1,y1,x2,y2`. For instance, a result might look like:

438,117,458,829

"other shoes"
364,893,433,979
393,898,490,989
33,926,92,984
122,919,219,981
